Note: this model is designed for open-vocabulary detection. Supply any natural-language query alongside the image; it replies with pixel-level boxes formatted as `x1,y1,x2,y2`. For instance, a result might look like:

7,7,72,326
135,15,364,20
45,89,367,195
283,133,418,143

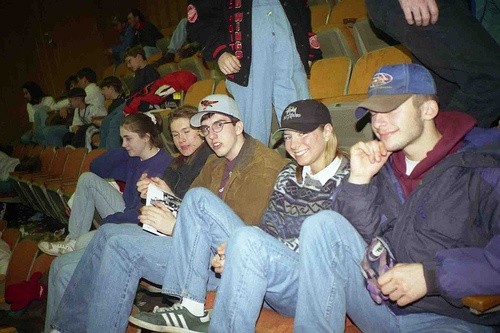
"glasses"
198,121,234,137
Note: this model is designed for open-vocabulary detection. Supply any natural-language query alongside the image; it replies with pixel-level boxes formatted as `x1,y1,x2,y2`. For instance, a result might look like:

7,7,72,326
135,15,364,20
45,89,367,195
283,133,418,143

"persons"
51,94,285,333
365,0,500,128
63,86,95,149
293,62,500,333
31,67,108,148
103,15,134,66
20,80,55,148
45,107,215,332
98,45,160,152
128,9,163,59
37,114,174,257
127,100,352,333
186,1,325,148
0,1,200,333
64,76,129,151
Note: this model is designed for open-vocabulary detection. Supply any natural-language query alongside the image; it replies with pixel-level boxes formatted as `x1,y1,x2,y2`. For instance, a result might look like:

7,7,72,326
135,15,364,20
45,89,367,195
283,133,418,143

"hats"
190,94,242,127
270,99,331,147
355,63,435,120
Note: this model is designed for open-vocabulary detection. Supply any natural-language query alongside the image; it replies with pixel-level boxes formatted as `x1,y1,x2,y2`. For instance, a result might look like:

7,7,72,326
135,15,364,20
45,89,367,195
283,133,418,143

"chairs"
0,0,500,333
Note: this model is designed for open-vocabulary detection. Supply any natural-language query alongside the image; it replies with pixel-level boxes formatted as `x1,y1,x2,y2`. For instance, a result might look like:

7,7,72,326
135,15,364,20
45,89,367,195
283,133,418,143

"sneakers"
129,303,210,333
38,235,76,257
